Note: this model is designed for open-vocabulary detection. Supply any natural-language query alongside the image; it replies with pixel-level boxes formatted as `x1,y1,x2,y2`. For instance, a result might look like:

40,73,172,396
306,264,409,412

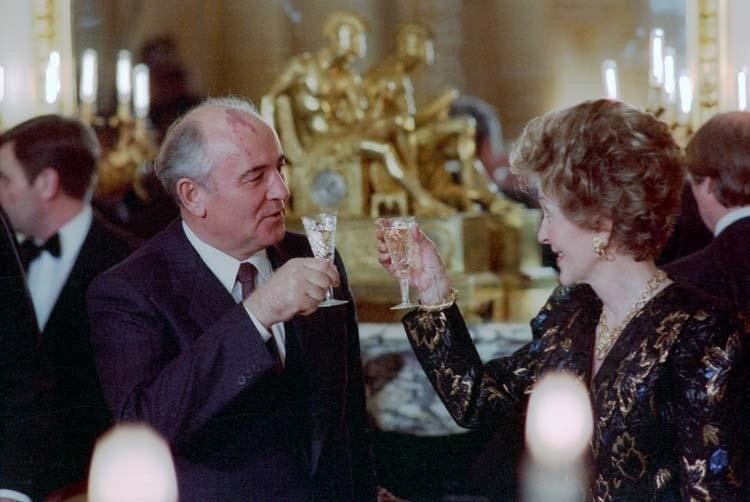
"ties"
236,263,255,299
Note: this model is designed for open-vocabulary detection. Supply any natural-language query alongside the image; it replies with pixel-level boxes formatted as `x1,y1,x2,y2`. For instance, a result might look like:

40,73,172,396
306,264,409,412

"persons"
376,99,749,502
89,95,398,501
262,10,455,219
1,207,44,499
656,104,748,329
658,171,720,266
359,24,496,213
1,114,147,500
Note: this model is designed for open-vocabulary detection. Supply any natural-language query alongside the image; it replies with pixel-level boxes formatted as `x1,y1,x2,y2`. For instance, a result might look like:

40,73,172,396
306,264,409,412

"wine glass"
376,215,421,309
300,210,350,308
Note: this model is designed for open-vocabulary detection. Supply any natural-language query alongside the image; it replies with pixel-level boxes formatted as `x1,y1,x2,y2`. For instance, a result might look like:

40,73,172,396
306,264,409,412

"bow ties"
19,232,60,261
